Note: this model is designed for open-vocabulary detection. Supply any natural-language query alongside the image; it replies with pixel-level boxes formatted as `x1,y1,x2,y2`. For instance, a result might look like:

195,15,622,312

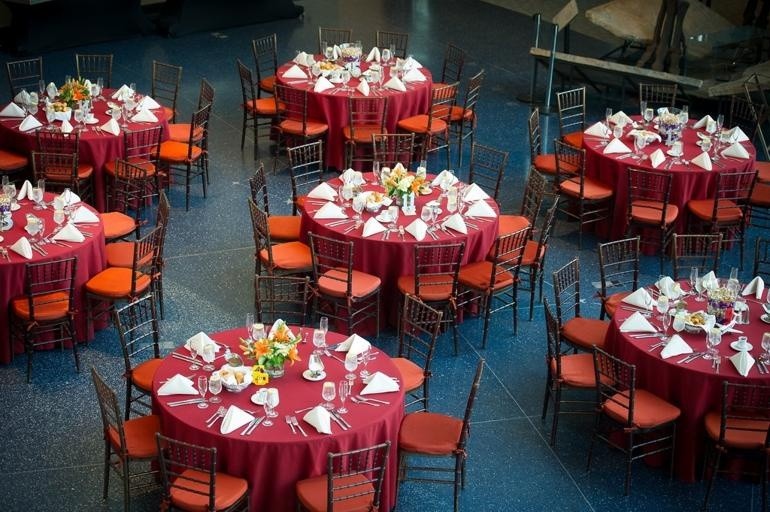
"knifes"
647,341,667,353
617,305,653,321
629,333,670,338
677,352,705,364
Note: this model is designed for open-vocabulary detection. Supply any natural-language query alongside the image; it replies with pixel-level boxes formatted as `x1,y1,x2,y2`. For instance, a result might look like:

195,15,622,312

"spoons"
752,356,769,374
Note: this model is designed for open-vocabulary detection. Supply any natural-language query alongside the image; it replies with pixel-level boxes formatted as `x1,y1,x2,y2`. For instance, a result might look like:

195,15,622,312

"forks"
712,354,721,374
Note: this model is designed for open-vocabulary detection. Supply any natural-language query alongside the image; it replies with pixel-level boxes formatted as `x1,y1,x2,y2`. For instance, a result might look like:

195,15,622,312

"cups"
702,328,721,359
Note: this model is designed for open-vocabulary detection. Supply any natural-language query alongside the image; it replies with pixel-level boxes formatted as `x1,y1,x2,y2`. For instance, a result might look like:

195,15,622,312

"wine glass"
158,313,399,437
646,267,707,340
307,160,497,241
1,76,162,137
0,175,98,262
284,40,427,98
585,101,750,171
730,267,770,365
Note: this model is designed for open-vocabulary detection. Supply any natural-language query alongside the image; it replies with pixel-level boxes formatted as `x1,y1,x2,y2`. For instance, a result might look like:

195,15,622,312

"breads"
220,368,245,385
692,313,705,326
49,103,66,113
321,62,335,69
369,191,384,205
